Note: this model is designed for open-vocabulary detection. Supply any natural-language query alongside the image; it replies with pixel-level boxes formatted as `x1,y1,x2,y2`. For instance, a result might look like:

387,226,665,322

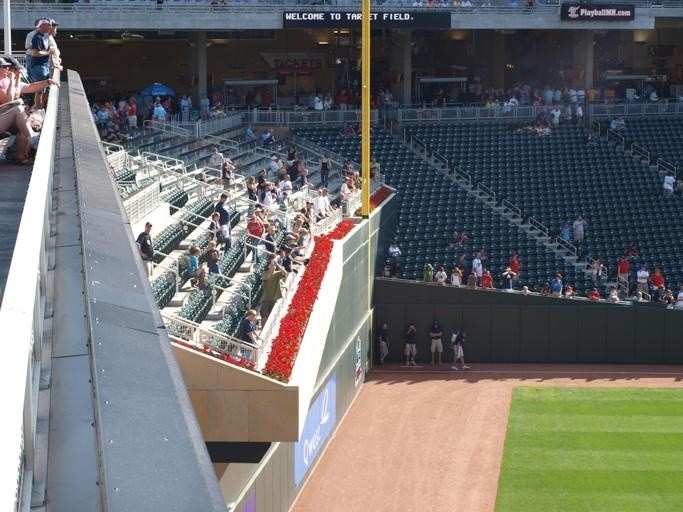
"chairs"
100,97,683,356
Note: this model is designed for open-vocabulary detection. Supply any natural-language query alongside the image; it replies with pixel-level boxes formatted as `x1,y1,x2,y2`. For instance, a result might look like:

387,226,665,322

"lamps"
69,34,75,39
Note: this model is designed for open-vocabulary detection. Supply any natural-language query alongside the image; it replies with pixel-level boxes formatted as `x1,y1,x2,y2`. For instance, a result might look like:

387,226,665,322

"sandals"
15,155,34,165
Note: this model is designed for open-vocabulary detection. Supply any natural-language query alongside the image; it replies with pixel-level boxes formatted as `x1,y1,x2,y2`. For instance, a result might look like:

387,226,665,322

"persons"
404,320,417,366
380,231,521,291
663,171,682,199
1,16,64,165
377,323,389,365
451,328,471,370
603,80,661,132
541,215,683,310
418,81,601,136
136,126,387,361
91,87,274,146
430,321,444,366
310,84,394,120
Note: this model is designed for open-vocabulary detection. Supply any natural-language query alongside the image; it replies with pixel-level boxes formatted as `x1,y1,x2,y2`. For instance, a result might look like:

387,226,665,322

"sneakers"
451,367,458,370
463,366,470,369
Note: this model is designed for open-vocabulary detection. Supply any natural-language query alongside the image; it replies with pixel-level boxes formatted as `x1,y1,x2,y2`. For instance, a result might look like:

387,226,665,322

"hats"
0,57,23,70
322,188,329,192
35,19,59,28
